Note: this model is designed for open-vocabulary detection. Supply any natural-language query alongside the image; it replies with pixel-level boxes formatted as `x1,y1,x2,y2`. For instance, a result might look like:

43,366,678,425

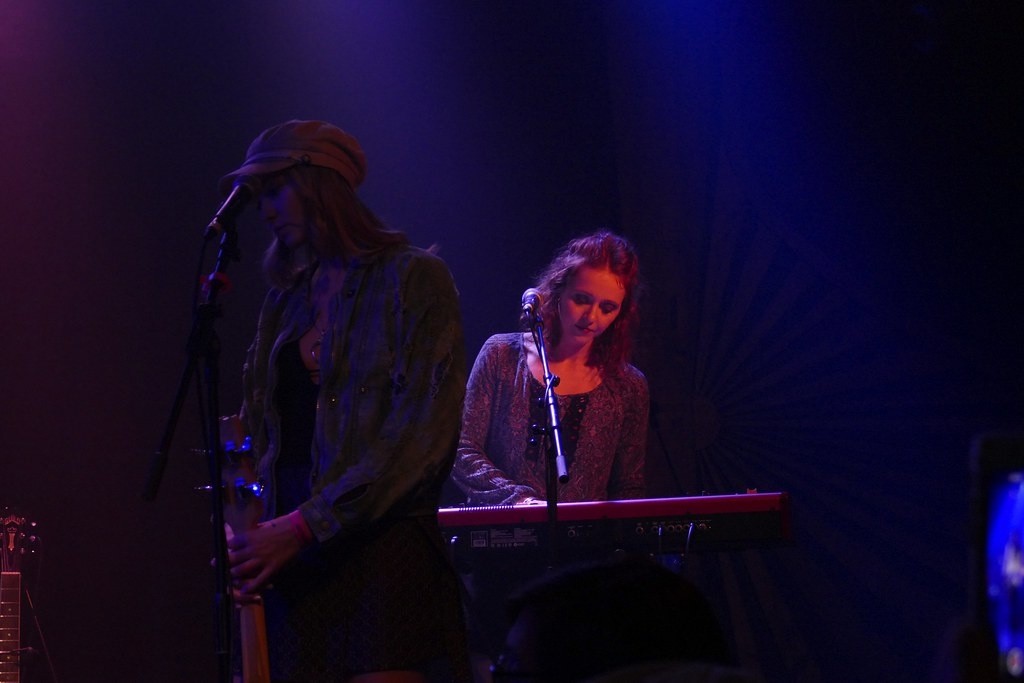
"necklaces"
308,314,330,366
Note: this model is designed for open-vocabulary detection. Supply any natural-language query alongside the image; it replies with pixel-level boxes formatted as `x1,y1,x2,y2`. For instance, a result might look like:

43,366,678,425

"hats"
221,120,365,190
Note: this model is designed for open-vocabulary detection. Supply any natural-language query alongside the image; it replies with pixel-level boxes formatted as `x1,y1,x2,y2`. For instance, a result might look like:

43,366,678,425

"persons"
450,231,655,681
200,116,476,681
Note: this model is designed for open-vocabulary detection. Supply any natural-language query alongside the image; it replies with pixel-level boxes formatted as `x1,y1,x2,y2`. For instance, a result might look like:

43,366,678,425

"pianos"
432,491,793,682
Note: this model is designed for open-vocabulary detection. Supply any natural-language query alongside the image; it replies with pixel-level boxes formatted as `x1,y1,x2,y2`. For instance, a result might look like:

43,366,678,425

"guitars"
196,414,275,683
1,512,47,683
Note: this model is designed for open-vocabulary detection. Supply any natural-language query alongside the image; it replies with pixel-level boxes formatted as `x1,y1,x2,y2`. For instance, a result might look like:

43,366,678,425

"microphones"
522,288,542,313
202,176,257,241
